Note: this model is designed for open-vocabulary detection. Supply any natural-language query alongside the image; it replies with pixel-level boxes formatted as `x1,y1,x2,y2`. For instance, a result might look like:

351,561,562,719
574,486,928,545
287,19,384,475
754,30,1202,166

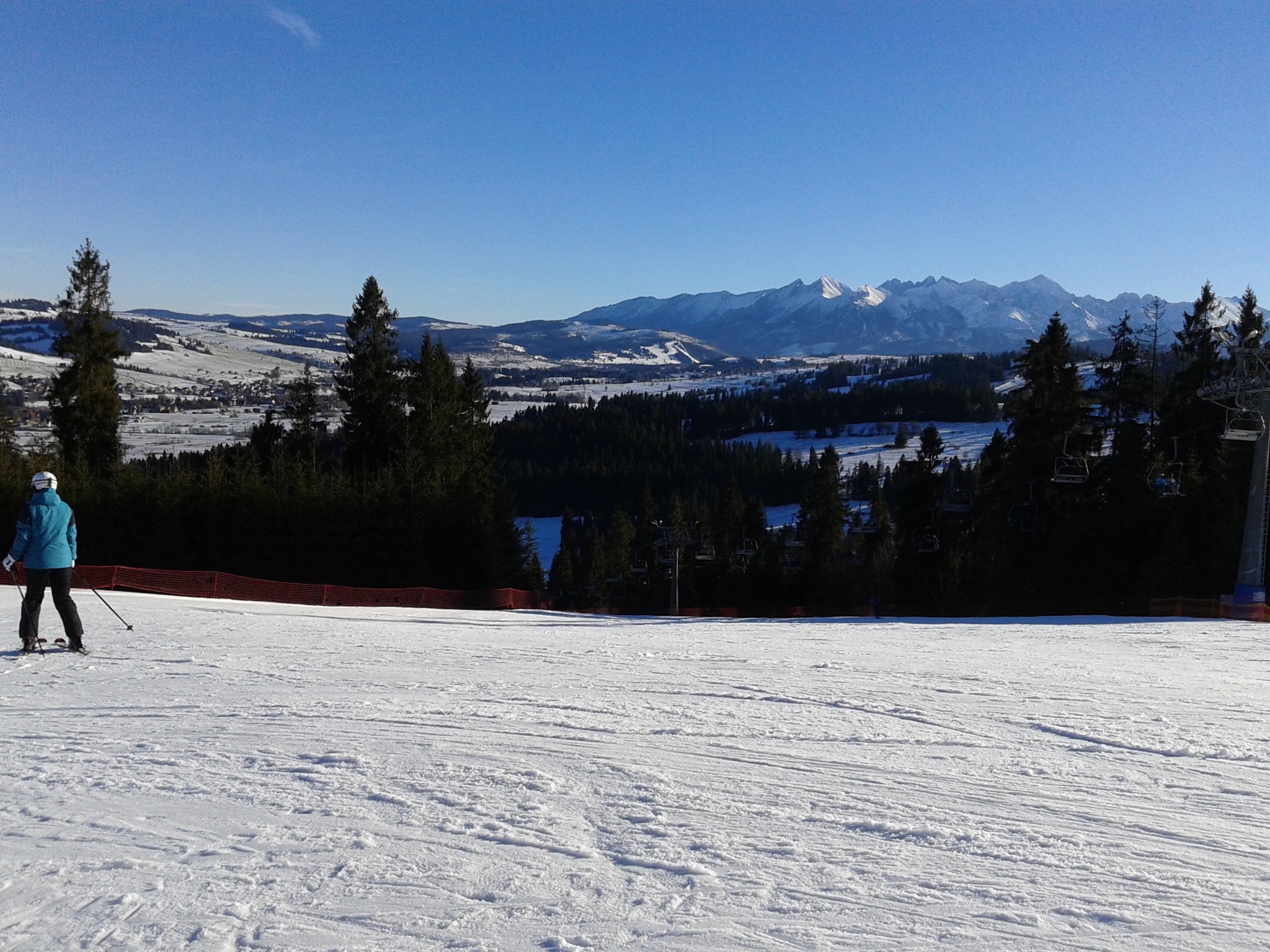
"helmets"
31,471,58,491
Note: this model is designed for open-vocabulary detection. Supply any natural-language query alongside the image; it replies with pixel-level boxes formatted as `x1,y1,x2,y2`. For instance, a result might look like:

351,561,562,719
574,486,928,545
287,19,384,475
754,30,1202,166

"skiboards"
4,637,94,663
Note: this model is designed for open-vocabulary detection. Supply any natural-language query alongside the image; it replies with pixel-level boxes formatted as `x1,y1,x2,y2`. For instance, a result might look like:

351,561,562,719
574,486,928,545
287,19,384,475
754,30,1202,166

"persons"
3,471,84,651
1156,472,1181,496
921,533,939,550
671,528,683,539
666,568,672,576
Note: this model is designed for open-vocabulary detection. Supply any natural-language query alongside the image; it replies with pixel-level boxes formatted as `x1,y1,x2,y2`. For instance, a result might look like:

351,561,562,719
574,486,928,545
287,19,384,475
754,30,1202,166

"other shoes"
23,637,35,652
68,636,82,649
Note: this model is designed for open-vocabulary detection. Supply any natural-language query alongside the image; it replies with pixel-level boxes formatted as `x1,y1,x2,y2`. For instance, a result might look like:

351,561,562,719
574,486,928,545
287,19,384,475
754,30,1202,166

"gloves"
2,554,15,572
